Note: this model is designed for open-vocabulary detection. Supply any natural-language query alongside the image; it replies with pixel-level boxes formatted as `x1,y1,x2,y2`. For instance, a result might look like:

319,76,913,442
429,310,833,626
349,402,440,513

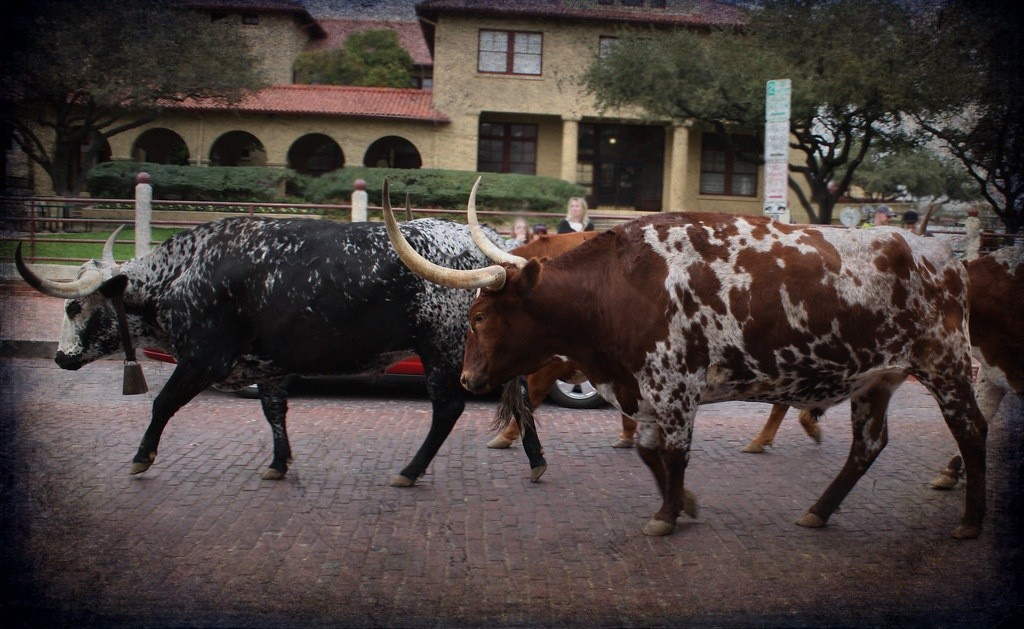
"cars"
140,346,608,407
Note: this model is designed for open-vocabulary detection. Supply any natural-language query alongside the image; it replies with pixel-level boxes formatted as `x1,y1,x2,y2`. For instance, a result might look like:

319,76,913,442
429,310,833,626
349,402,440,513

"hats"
877,203,896,216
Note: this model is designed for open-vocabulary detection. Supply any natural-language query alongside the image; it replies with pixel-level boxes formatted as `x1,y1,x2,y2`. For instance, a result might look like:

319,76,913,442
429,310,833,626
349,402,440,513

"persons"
861,204,935,238
558,197,594,234
504,216,548,250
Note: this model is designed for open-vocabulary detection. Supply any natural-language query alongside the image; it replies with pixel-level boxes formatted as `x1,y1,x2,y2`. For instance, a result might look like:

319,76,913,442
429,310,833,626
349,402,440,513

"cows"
380,171,1023,542
15,225,547,488
